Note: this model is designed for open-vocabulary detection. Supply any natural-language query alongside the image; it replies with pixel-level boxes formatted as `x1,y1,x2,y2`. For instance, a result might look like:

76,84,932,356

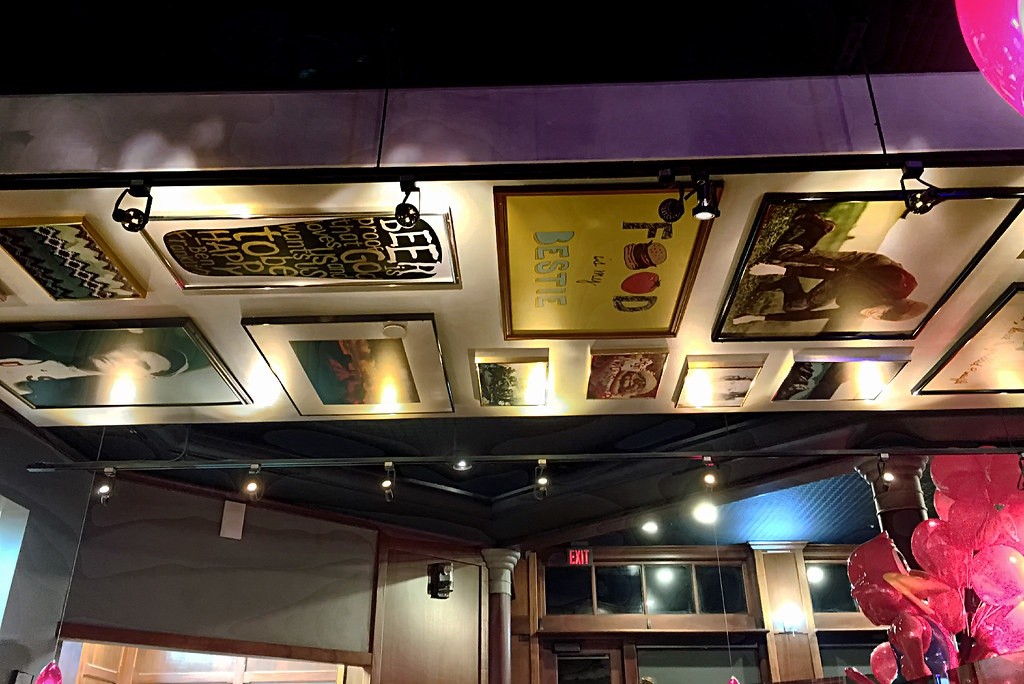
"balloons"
869,454,1024,684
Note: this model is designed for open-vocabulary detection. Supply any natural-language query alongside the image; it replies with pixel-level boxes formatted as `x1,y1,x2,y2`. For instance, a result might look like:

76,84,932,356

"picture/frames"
493,179,725,342
240,312,455,417
0,316,254,411
769,346,914,402
711,187,1024,342
671,353,769,409
911,282,1024,396
0,216,147,307
585,347,671,400
474,348,549,407
139,207,463,296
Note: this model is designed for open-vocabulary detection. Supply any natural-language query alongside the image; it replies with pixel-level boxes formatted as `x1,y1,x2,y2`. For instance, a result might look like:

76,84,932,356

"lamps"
381,462,397,502
684,174,724,221
874,453,895,498
535,459,549,501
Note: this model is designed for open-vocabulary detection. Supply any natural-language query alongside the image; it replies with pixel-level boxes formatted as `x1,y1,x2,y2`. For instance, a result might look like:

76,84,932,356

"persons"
328,339,380,404
733,216,931,326
